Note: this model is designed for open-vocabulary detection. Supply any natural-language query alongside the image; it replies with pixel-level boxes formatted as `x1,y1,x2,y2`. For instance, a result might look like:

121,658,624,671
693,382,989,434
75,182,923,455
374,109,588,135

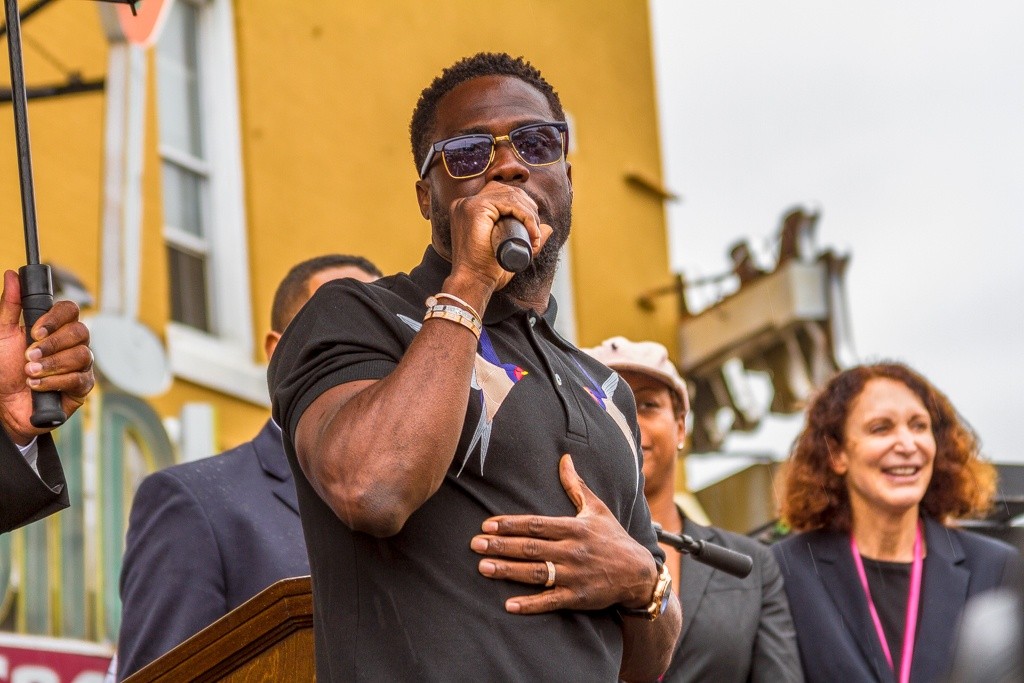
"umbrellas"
0,0,144,425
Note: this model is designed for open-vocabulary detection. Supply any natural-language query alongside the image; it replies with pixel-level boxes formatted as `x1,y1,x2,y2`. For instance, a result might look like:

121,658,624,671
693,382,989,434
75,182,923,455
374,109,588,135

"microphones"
489,214,533,273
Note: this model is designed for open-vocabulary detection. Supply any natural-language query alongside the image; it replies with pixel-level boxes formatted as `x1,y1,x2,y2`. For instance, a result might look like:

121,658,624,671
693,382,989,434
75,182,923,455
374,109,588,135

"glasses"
421,120,569,182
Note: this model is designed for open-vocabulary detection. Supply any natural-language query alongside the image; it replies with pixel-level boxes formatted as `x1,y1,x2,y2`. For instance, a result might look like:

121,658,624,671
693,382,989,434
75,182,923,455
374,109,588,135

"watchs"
614,556,672,623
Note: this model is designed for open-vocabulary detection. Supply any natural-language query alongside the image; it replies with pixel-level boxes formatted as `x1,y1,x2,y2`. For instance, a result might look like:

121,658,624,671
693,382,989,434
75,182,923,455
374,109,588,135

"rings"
544,560,556,586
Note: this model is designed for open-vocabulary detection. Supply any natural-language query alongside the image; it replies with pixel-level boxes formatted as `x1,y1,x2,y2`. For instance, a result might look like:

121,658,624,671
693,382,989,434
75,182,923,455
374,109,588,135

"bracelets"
423,293,482,341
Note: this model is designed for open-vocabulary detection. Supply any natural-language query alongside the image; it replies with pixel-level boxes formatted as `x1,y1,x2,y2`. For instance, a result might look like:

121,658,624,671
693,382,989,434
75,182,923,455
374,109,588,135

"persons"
765,361,1024,682
579,332,804,682
0,267,95,537
114,254,385,683
265,51,685,683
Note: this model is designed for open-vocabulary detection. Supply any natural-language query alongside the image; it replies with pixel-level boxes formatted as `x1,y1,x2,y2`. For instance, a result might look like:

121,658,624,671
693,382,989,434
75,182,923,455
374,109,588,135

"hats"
580,335,691,417
